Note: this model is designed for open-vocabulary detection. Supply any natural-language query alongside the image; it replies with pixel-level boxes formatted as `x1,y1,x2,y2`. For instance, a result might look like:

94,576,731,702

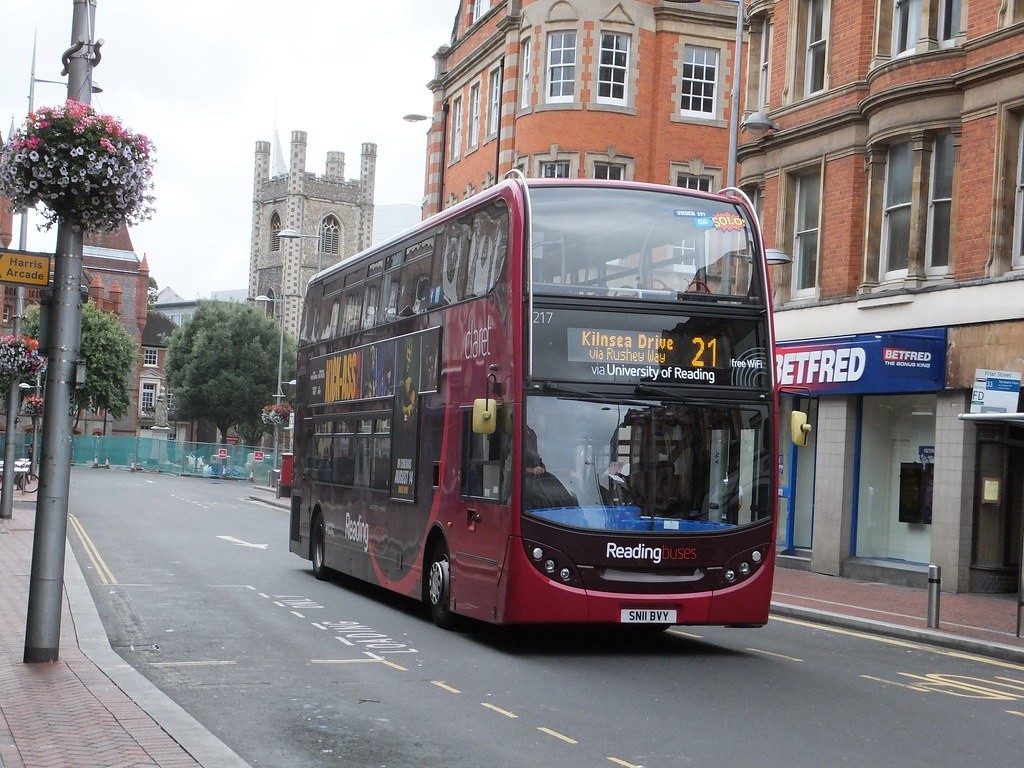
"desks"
0,467,30,495
0,461,32,490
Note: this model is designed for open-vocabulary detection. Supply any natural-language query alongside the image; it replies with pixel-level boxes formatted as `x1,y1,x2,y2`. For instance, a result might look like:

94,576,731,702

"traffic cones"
220,462,228,480
246,470,256,483
129,459,135,473
102,457,111,469
92,455,101,468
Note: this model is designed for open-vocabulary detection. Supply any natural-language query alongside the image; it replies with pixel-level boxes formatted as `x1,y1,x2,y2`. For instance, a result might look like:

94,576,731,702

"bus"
288,168,812,629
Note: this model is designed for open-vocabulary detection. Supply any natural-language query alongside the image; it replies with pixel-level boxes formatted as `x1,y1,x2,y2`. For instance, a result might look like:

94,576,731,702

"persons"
503,426,578,479
616,461,675,514
28,442,33,460
722,469,743,522
156,387,168,427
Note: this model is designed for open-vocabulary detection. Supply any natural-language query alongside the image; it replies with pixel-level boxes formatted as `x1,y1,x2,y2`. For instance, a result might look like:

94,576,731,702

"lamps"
742,110,781,136
402,113,435,123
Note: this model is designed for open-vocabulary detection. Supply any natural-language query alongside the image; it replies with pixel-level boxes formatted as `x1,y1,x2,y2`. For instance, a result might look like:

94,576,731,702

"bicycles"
0,457,38,493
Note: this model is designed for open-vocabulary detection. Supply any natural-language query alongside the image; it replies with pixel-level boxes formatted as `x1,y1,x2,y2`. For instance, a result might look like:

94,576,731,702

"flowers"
0,333,48,377
20,393,43,417
0,96,160,241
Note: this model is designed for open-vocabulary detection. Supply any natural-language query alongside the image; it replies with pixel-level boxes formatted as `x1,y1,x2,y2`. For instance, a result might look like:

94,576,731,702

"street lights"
254,296,296,488
662,1,793,524
19,371,42,481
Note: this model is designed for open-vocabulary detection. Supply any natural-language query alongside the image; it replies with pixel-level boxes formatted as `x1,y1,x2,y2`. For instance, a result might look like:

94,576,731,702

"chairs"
329,306,395,339
312,456,389,489
612,278,716,303
